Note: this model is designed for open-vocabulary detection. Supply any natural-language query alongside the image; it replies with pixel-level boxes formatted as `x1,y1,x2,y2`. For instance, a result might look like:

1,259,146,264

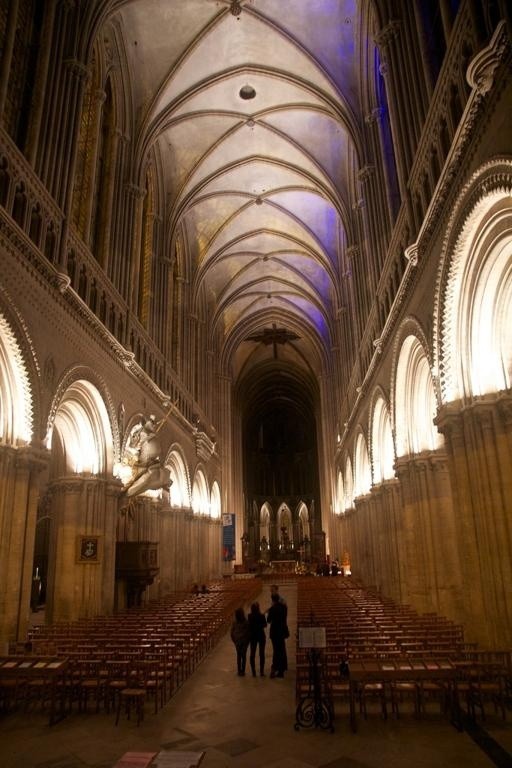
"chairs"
0,584,229,727
296,595,511,730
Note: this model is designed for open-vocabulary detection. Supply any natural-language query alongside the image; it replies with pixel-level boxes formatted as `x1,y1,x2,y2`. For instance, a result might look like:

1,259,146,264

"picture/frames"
76,535,102,563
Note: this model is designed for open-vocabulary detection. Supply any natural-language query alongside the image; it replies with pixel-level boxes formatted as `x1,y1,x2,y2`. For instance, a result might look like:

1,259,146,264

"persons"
330,561,337,575
228,607,249,676
266,592,290,679
200,585,209,595
190,584,200,596
269,583,288,610
321,559,330,576
315,563,322,577
246,600,267,677
